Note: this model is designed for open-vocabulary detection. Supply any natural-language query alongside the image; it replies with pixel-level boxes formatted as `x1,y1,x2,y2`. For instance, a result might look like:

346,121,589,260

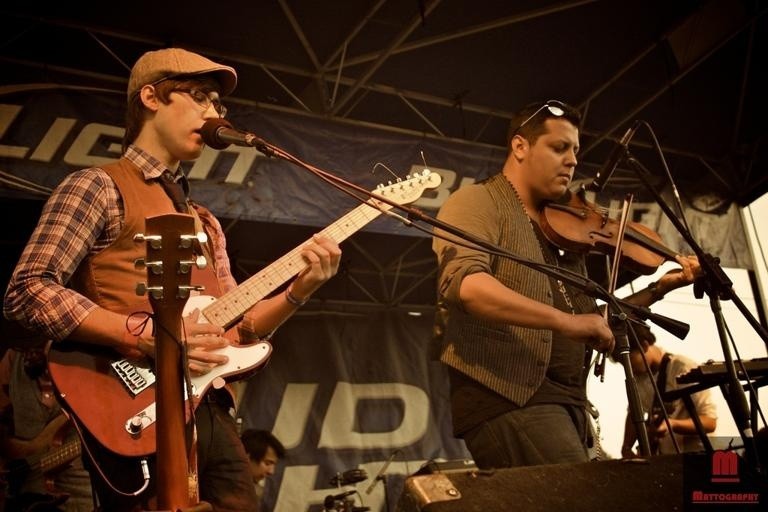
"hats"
127,47,238,101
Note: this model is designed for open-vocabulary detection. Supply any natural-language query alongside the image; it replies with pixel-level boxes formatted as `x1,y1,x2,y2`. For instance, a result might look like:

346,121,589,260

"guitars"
44,169,441,458
636,405,674,455
1,415,81,496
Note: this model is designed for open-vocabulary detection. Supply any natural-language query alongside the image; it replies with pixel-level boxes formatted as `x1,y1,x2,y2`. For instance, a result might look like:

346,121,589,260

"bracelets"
123,315,145,351
621,447,632,452
648,282,664,301
285,284,311,306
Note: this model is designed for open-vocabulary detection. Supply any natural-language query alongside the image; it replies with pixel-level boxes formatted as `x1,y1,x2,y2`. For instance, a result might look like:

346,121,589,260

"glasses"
170,87,228,119
515,99,566,133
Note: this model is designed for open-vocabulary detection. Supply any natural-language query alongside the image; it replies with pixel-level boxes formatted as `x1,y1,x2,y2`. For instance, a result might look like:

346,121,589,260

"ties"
160,178,204,256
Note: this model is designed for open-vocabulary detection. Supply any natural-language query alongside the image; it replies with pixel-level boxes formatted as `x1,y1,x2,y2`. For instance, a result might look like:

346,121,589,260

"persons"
0,347,96,511
239,427,287,485
0,47,343,512
608,318,719,459
431,97,705,473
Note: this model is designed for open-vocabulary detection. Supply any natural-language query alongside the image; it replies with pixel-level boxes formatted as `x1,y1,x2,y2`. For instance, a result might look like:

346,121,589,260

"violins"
540,191,683,273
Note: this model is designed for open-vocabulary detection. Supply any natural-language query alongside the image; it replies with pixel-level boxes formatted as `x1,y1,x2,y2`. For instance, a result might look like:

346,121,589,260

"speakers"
396,444,768,512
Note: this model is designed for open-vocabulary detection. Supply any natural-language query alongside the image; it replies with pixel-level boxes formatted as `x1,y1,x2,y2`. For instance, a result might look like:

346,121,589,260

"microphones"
201,117,293,163
589,120,640,193
